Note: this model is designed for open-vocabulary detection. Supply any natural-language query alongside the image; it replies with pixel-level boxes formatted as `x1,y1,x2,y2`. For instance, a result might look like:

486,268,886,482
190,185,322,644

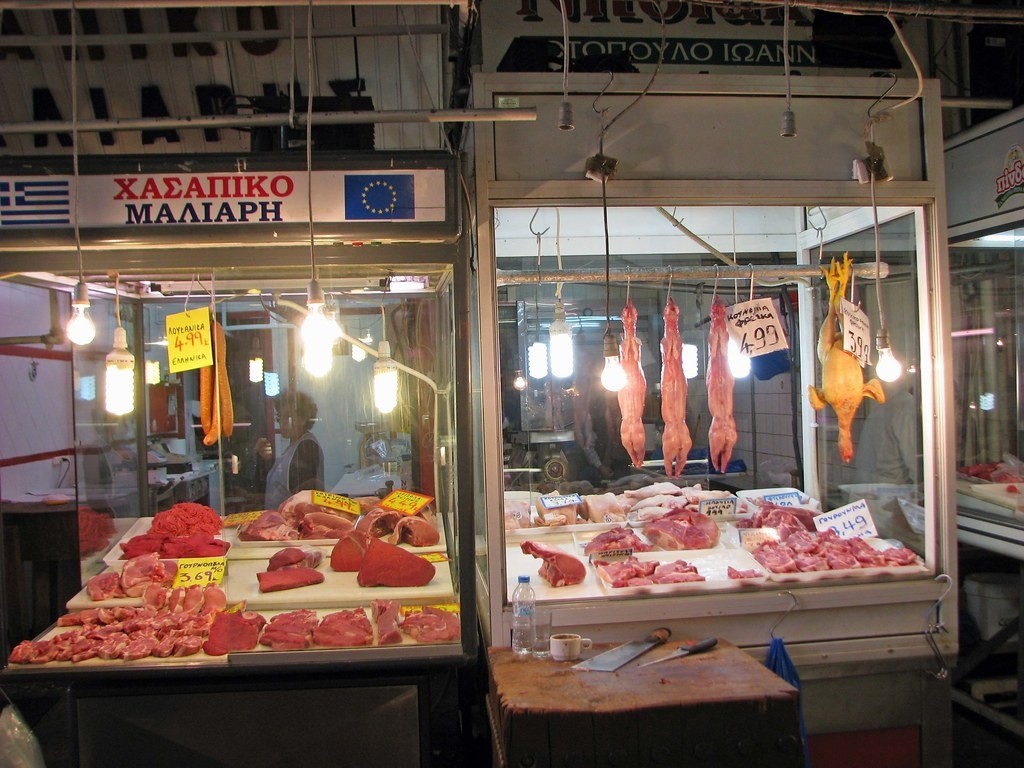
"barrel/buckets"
962,572,1023,643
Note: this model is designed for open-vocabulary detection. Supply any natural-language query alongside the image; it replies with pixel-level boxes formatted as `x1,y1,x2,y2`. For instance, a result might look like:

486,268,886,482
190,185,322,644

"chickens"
807,250,886,465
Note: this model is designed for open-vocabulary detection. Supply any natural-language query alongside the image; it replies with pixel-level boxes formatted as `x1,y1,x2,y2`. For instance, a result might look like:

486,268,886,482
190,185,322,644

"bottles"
512,575,536,655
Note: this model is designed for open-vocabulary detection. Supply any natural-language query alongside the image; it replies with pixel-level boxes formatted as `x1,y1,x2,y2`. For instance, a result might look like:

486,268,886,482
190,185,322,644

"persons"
255,392,324,510
84,409,141,518
574,358,630,486
855,385,915,484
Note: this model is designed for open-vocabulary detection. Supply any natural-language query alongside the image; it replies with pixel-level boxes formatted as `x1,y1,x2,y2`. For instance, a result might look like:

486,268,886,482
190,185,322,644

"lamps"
548,208,573,379
351,294,398,415
105,278,135,415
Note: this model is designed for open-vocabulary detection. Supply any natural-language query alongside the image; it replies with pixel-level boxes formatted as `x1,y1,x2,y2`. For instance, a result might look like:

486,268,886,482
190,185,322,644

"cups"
532,605,551,656
550,634,593,661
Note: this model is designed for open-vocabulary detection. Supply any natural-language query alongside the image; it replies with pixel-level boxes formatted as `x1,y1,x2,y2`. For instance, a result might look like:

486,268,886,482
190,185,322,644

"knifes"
642,637,718,666
570,627,672,672
